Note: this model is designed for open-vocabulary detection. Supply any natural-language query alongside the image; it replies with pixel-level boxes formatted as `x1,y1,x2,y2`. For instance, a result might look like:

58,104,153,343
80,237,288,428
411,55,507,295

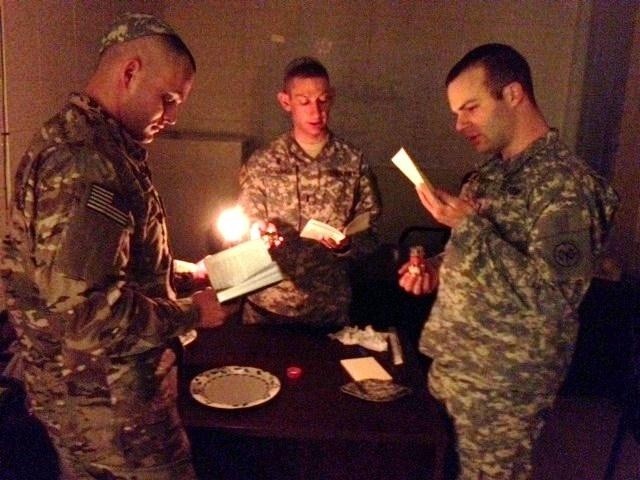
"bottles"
409,246,425,277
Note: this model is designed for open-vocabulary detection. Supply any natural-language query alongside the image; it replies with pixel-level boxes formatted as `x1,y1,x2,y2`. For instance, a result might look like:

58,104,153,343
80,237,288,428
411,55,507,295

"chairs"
386,224,448,323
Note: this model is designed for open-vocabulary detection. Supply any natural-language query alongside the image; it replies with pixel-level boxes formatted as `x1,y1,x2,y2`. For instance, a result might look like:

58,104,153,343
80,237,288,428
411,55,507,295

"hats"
96,11,179,55
286,55,322,72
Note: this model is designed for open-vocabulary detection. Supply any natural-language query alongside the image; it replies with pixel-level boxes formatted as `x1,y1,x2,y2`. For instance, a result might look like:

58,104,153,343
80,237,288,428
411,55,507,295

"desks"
177,323,452,476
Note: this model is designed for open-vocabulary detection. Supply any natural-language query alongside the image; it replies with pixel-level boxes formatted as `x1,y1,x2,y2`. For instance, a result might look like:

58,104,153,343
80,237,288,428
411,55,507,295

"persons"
395,41,620,479
2,10,242,479
239,57,382,323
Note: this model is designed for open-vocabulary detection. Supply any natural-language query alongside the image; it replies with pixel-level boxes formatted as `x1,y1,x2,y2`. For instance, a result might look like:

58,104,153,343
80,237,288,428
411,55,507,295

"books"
202,238,282,304
389,148,435,194
300,211,370,244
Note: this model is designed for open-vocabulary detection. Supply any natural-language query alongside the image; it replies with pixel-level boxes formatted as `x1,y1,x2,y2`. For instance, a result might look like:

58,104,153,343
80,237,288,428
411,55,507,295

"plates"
190,364,282,411
